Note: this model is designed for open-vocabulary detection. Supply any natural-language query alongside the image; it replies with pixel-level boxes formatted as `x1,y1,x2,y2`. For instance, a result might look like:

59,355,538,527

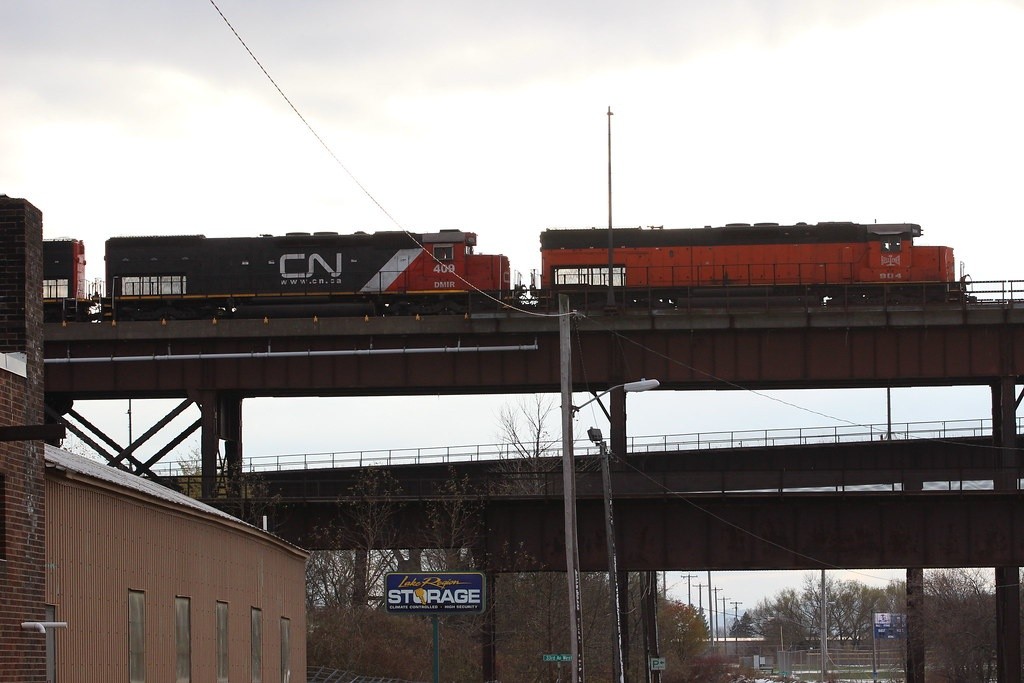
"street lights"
587,425,625,683
559,379,661,683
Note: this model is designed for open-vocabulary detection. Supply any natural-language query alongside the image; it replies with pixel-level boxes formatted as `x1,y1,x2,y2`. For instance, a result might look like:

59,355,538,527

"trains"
36,217,979,306
